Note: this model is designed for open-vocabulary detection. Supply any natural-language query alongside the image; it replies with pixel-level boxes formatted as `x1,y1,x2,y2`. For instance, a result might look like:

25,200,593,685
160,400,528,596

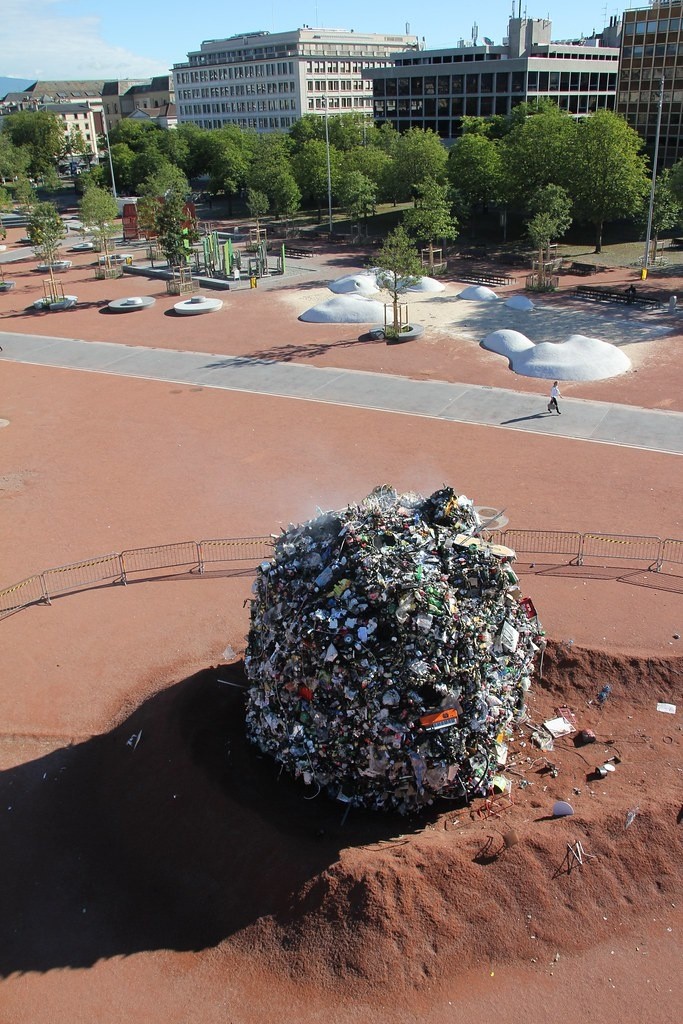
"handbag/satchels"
548,401,555,409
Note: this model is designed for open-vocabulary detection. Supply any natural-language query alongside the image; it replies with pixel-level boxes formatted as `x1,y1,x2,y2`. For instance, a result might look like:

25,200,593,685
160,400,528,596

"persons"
625,285,636,304
548,381,564,414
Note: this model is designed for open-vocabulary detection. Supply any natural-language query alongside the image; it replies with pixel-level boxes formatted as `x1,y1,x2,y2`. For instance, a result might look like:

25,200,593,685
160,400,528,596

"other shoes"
557,411,561,414
548,409,552,413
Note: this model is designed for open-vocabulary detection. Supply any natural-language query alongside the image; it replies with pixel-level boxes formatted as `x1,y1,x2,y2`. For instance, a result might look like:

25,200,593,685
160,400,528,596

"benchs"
568,261,596,275
570,285,660,309
280,246,314,258
456,270,516,286
